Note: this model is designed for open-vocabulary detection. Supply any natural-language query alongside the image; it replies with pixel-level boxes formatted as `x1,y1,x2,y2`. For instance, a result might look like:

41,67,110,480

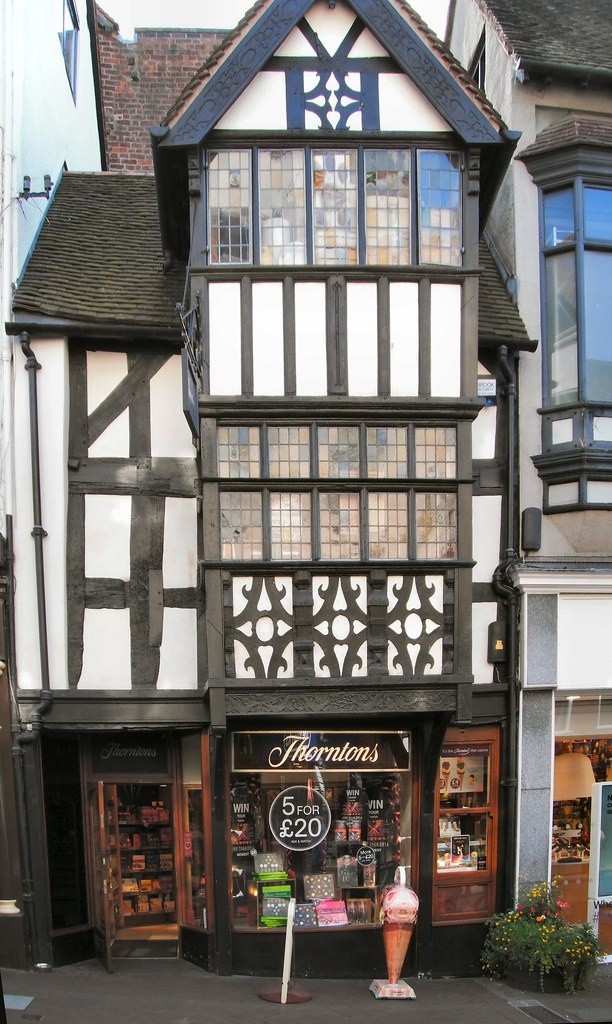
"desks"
339,885,380,923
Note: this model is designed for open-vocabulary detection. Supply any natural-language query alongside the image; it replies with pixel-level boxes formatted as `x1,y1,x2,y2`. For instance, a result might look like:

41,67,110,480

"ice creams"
442,762,450,792
382,887,419,984
456,758,465,791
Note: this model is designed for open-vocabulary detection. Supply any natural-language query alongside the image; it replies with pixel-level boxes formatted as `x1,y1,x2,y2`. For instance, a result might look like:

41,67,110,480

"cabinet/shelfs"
437,839,486,873
246,879,296,930
104,820,177,926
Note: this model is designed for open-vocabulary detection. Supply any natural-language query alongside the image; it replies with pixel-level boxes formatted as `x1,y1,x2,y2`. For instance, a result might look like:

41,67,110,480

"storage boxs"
109,798,176,915
253,788,394,926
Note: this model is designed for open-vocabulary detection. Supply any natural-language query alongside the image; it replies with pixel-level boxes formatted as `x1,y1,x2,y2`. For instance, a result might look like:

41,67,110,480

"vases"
505,945,581,993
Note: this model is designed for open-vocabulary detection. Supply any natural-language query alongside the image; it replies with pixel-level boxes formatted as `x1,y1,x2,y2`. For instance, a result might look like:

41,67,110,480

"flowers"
479,873,612,998
